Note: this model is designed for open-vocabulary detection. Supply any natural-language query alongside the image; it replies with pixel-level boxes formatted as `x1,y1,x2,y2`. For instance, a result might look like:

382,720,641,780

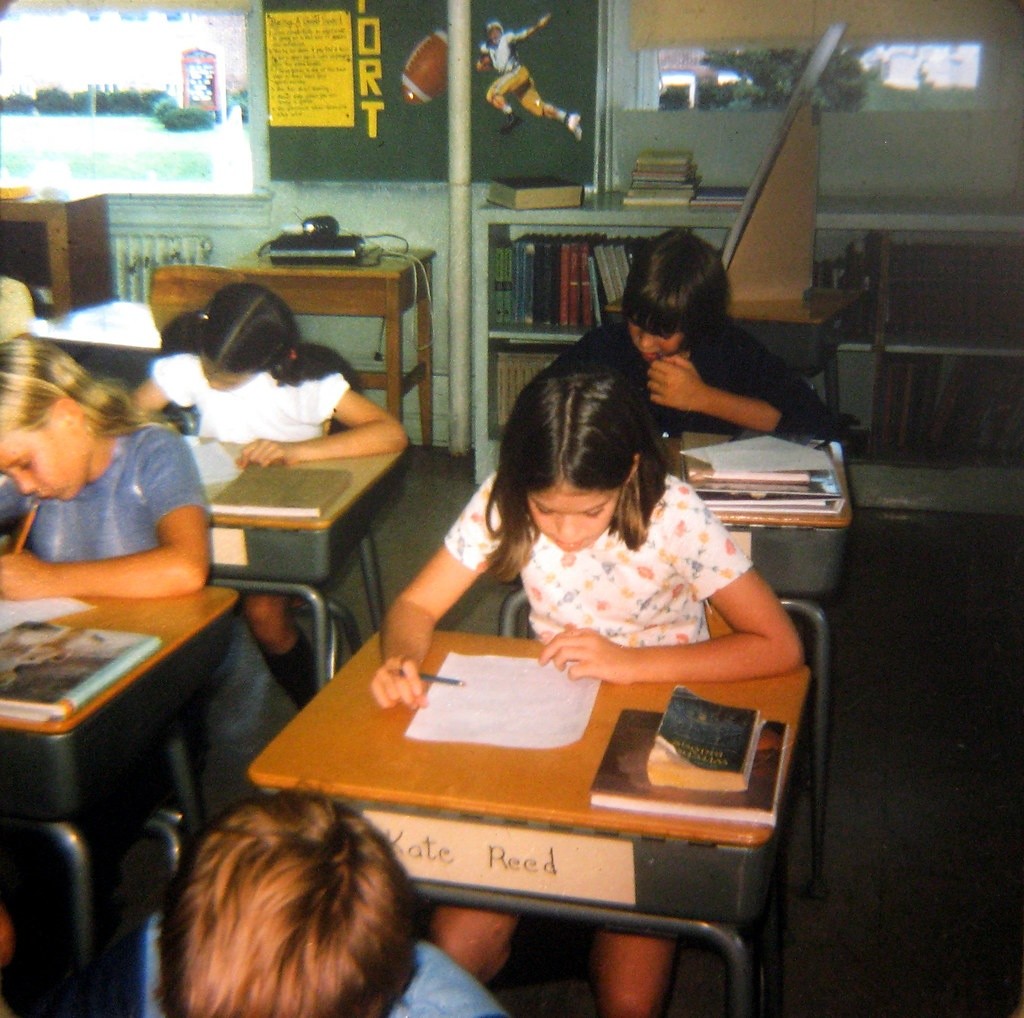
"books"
484,173,584,211
809,247,1023,342
590,684,791,829
1,622,161,725
208,460,354,518
491,230,642,328
674,354,1024,516
623,148,749,207
490,343,566,438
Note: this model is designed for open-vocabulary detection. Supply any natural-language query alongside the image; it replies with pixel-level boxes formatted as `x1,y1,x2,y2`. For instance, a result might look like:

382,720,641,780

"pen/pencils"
13,498,41,556
388,668,467,689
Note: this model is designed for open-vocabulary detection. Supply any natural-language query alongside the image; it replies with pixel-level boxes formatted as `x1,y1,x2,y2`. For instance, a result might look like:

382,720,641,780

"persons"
367,354,806,1018
570,227,831,436
0,338,212,781
477,12,583,141
34,787,513,1018
126,279,411,711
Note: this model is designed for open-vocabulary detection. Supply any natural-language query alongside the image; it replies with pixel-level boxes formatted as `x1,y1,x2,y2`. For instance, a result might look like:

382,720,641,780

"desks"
247,620,811,1018
496,427,855,892
156,244,433,448
181,434,398,656
0,584,238,991
0,193,112,317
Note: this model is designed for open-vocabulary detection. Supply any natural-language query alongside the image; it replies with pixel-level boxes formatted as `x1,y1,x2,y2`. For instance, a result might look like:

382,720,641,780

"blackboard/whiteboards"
262,1,606,196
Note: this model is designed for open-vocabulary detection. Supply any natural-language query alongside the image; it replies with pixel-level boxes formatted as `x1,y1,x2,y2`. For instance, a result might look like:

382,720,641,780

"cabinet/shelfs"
472,196,1024,519
